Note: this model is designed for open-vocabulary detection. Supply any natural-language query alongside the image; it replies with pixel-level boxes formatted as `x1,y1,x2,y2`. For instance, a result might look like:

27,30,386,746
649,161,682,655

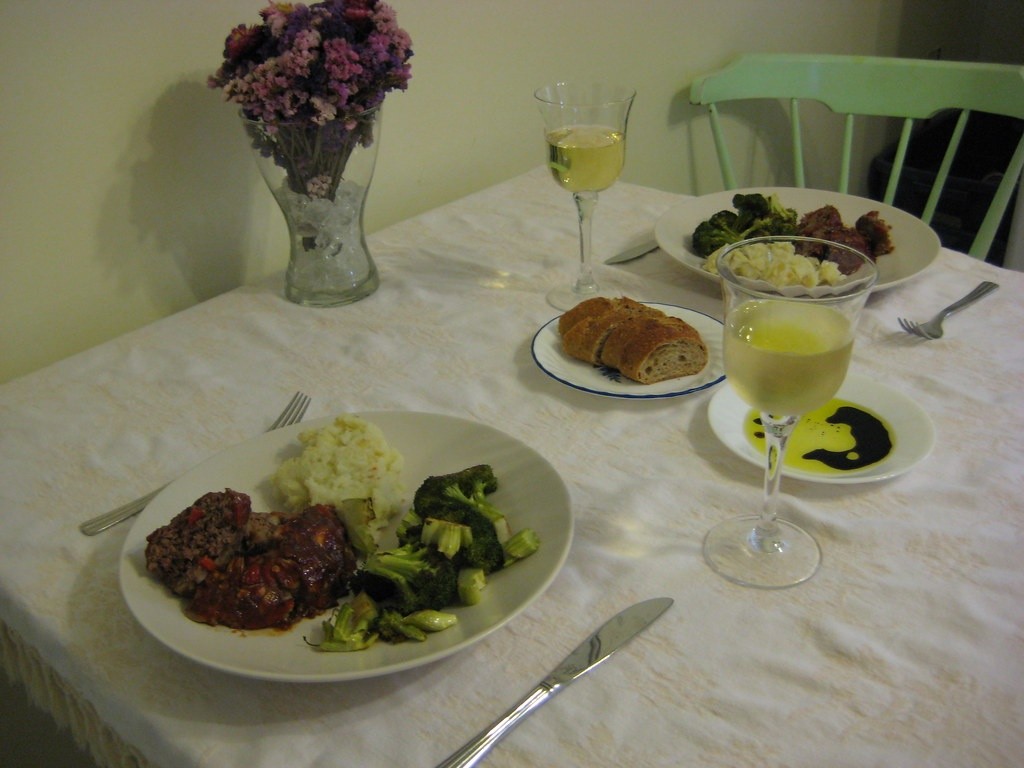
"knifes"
435,597,673,768
605,238,662,265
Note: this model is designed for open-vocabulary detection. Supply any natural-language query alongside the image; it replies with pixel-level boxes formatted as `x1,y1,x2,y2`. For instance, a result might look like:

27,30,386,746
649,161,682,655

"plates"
707,372,937,483
118,410,577,683
531,301,727,398
655,186,942,294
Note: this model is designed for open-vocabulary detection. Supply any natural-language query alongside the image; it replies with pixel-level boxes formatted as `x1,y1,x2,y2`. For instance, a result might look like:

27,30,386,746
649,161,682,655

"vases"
237,105,383,310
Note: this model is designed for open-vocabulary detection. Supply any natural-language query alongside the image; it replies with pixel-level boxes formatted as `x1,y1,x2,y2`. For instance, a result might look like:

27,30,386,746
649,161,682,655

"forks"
898,281,1000,340
80,391,310,537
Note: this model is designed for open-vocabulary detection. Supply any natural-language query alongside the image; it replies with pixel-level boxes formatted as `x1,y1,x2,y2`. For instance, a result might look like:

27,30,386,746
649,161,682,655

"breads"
559,296,709,383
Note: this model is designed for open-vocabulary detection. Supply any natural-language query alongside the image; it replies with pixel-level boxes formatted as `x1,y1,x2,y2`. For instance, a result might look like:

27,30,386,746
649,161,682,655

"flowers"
203,0,415,197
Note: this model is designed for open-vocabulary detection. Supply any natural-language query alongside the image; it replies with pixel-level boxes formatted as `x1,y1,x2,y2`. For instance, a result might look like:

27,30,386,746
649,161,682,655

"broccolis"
691,193,799,258
299,463,540,650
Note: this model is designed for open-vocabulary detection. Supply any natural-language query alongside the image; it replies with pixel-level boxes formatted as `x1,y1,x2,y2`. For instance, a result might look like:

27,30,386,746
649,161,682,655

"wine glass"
535,80,638,312
702,235,879,588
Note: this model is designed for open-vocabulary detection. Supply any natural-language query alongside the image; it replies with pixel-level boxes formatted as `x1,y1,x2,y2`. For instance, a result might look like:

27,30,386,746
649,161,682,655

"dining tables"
0,162,1024,768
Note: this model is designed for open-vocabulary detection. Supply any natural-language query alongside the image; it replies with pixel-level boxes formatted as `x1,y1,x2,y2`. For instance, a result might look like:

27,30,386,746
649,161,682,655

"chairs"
690,51,1024,274
